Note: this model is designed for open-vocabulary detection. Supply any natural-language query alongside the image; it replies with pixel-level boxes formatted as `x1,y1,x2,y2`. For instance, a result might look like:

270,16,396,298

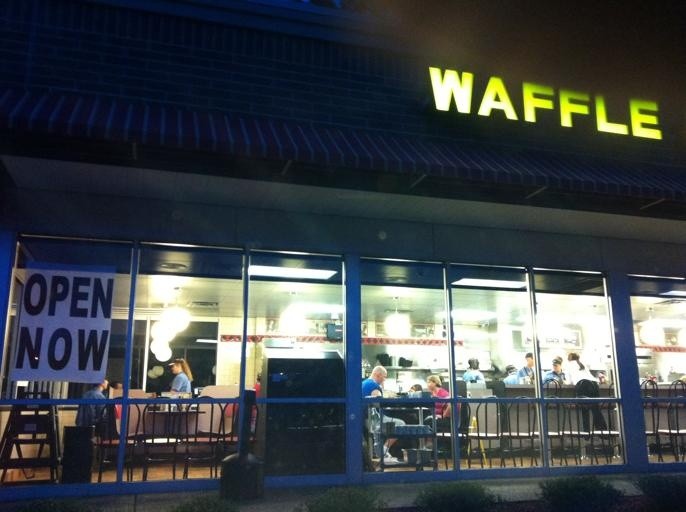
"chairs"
2,392,62,484
440,379,685,469
95,395,258,482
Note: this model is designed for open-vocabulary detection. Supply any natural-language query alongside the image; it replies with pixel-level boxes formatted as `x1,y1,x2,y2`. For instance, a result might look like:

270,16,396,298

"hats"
427,375,442,387
169,359,183,366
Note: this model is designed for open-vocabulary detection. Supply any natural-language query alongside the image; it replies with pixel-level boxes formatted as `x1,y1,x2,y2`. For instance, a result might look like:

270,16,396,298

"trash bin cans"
63,426,95,484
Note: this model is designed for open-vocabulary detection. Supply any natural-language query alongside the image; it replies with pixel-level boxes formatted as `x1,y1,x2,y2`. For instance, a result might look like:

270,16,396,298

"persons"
75,379,113,464
462,352,608,441
423,375,460,451
168,358,193,393
362,366,405,458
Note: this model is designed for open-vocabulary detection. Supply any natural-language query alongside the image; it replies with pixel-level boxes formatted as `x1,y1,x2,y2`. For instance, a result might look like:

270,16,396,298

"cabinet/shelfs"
378,395,437,473
